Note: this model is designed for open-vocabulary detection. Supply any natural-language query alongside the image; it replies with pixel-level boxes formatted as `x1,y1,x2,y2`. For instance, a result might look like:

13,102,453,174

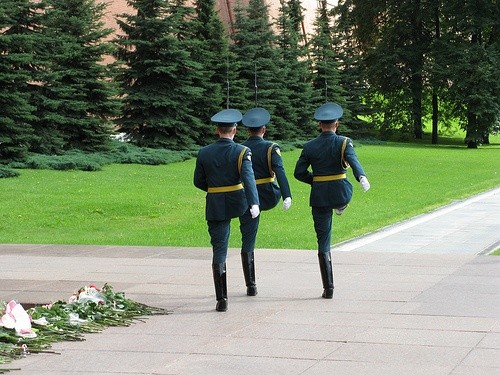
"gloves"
284,196,291,210
250,205,260,219
360,177,370,192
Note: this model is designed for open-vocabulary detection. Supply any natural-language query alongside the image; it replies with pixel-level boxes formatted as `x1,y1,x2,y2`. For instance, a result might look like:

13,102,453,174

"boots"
318,251,334,298
241,252,257,296
336,206,346,214
212,263,228,311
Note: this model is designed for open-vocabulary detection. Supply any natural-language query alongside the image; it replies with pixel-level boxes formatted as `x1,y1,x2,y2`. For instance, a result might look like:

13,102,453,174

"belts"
313,174,346,181
208,184,243,193
255,178,274,184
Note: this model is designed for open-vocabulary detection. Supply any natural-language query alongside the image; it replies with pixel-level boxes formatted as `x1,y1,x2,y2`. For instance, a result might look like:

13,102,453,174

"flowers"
0,284,170,374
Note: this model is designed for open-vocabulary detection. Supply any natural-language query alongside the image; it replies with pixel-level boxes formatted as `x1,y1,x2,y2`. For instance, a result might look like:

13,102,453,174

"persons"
293,102,370,299
238,108,292,296
193,109,260,311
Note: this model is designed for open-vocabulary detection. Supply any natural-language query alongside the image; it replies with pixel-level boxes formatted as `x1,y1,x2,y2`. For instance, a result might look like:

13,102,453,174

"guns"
325,59,327,103
226,57,231,109
254,62,257,108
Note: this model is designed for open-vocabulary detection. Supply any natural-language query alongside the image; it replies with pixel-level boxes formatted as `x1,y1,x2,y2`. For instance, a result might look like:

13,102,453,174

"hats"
313,102,343,123
211,109,242,125
242,108,270,128
